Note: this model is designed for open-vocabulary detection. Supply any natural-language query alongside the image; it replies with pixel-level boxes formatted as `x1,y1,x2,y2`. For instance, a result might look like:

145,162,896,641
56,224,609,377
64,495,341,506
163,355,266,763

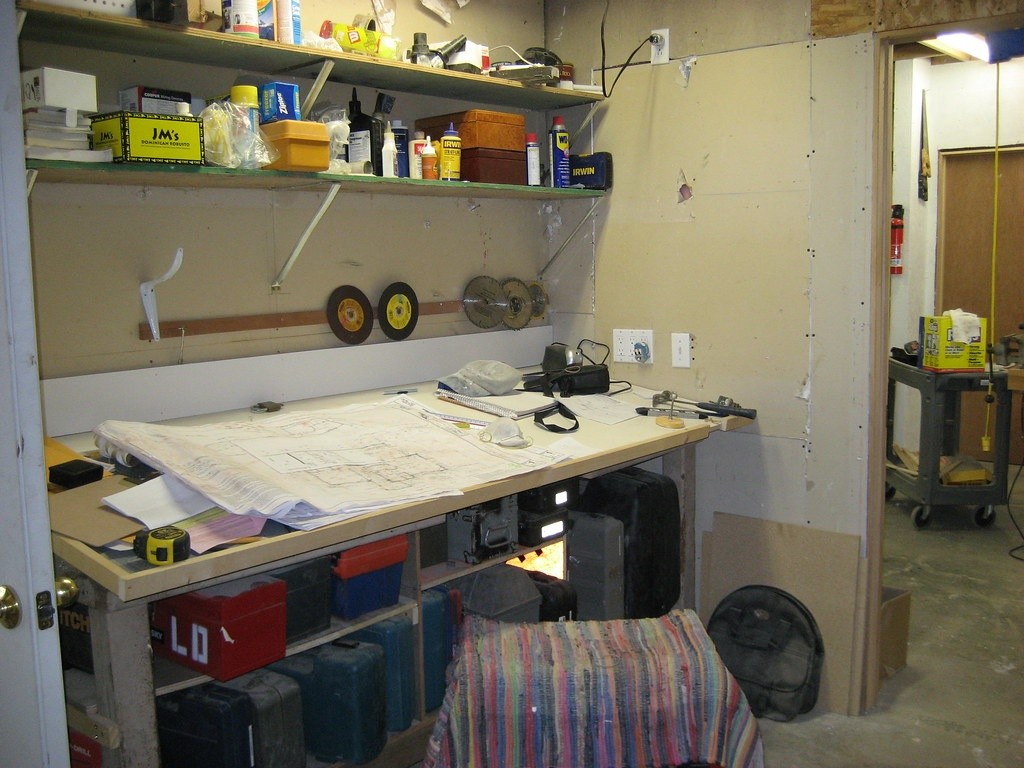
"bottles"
382,119,410,178
320,19,402,60
548,116,571,188
439,121,461,181
345,86,385,177
277,0,294,44
175,102,192,116
222,0,260,39
525,133,540,186
421,136,439,179
382,121,399,178
230,85,259,169
409,132,427,179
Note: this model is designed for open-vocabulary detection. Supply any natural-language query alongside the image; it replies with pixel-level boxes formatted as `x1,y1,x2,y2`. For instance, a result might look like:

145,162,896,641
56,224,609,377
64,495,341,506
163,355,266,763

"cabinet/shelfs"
16,0,603,293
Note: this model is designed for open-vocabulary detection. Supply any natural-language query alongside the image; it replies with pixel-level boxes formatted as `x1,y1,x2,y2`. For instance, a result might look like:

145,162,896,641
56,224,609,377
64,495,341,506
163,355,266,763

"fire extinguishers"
888,204,905,275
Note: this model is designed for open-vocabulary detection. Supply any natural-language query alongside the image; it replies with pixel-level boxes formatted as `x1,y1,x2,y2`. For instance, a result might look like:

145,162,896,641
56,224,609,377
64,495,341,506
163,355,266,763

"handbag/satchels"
557,364,610,398
706,584,825,722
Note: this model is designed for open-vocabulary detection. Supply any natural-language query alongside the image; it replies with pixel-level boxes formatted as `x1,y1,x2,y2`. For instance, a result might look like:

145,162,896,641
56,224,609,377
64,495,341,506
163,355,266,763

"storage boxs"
339,616,419,731
421,584,452,712
86,110,206,167
570,511,625,619
118,84,191,115
441,562,542,625
259,119,331,172
917,316,988,373
414,109,526,152
879,584,911,682
212,669,307,768
264,640,388,764
331,535,410,621
330,22,395,59
206,82,301,124
461,148,528,185
266,554,331,644
20,66,99,115
571,467,680,619
157,684,257,768
151,574,287,683
403,40,483,75
517,506,569,546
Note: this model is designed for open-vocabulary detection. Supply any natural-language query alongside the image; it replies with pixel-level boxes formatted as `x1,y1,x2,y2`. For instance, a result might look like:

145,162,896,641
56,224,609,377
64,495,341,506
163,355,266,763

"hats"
435,360,523,396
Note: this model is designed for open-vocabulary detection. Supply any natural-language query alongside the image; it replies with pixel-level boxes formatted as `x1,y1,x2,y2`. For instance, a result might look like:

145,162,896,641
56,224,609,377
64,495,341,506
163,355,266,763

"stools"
422,609,764,768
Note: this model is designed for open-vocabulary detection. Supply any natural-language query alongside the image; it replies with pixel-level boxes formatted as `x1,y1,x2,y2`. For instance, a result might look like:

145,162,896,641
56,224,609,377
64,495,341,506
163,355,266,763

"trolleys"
883,341,1012,530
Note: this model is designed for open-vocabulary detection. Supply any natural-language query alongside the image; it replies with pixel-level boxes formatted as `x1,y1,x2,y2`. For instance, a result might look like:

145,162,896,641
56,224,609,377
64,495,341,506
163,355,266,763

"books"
23,109,113,162
438,388,556,419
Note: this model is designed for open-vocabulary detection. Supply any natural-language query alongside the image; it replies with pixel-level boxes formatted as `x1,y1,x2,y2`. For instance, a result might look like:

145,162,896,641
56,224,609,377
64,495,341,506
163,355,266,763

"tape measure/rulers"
134,526,191,565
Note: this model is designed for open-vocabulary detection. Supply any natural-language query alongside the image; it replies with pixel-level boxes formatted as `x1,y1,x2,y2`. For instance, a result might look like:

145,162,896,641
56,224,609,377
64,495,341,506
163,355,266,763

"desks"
48,362,752,768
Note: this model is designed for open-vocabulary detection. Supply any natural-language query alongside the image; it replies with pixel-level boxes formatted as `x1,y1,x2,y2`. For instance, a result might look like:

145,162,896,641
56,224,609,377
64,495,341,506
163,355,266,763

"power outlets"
612,329,655,364
650,28,670,65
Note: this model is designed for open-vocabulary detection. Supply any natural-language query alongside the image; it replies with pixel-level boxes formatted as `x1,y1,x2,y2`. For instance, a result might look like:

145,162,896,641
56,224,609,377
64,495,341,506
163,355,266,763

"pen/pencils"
636,407,710,420
